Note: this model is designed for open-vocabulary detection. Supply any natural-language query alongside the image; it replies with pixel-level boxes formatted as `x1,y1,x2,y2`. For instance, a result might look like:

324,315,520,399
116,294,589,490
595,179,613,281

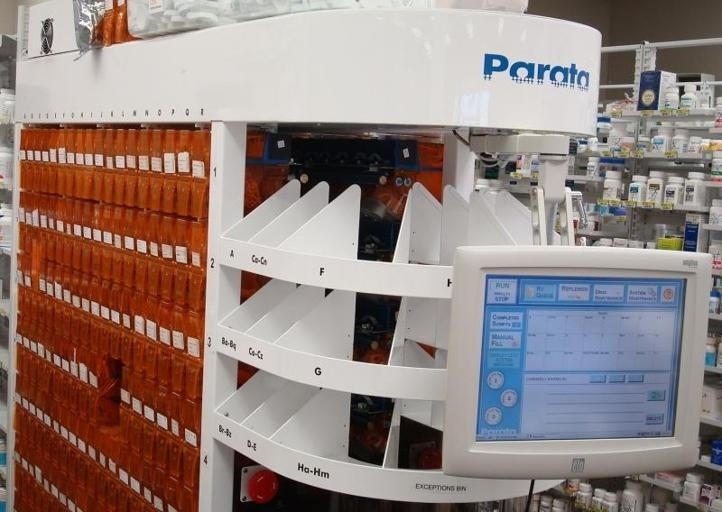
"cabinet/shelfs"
469,85,722,512
12,4,602,512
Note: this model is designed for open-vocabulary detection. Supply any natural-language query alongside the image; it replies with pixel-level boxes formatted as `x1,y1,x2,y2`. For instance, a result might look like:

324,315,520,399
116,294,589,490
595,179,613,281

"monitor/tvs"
442,245,713,479
